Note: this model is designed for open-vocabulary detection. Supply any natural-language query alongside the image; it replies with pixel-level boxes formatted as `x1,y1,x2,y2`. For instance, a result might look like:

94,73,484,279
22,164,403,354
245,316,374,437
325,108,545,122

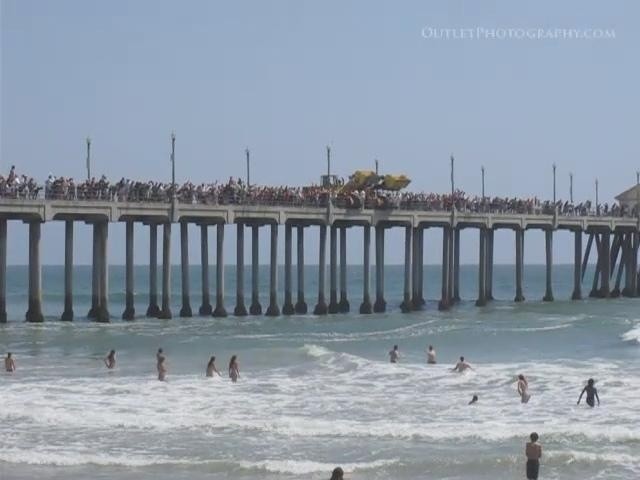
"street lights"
85,135,92,179
635,166,640,199
325,141,333,193
170,129,177,189
375,160,379,174
244,146,251,186
594,175,600,205
481,166,485,197
449,154,456,193
552,162,557,203
569,174,574,201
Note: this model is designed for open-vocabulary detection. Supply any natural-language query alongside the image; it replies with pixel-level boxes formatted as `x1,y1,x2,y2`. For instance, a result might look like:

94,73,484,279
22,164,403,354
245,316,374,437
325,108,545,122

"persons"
159,357,166,381
468,395,478,405
387,344,398,363
4,352,16,372
453,356,472,372
102,350,116,368
156,347,163,370
576,378,600,407
228,358,240,381
517,374,531,403
1,166,640,218
330,466,344,480
206,356,221,377
233,355,240,371
525,432,542,480
424,345,437,364
